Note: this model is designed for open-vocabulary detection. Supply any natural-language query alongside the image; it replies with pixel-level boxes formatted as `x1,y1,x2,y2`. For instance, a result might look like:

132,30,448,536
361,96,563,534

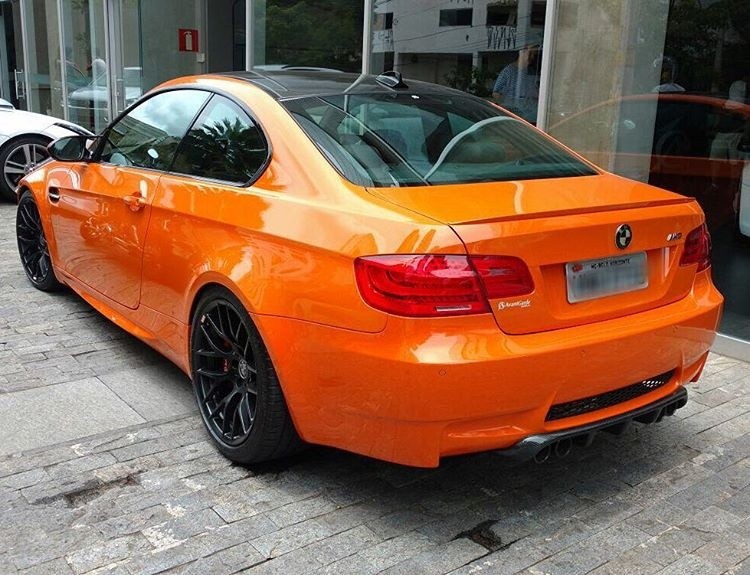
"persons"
64,140,80,158
492,48,537,120
86,48,106,127
658,48,750,160
55,47,78,124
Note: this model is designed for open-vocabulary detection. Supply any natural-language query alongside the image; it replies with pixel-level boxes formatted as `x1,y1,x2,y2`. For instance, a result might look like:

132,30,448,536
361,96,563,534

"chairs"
364,128,407,165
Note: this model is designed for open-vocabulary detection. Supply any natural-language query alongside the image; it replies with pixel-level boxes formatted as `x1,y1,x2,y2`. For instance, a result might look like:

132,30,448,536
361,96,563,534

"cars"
53,59,142,130
16,71,724,469
547,93,750,231
0,98,97,204
